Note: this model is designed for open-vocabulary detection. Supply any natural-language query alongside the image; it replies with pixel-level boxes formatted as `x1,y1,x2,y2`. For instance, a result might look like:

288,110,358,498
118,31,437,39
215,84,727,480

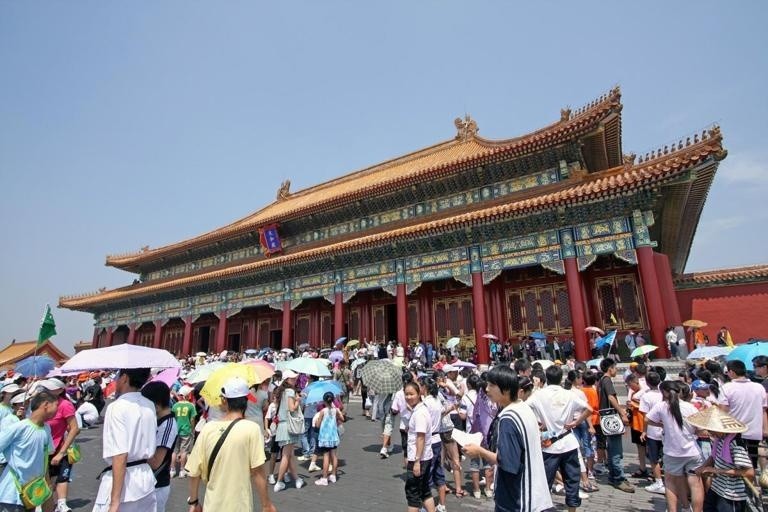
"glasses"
752,363,764,368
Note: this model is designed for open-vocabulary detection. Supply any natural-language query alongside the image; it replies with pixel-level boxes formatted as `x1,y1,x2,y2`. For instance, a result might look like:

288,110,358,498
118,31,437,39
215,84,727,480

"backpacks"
704,334,709,343
485,405,502,454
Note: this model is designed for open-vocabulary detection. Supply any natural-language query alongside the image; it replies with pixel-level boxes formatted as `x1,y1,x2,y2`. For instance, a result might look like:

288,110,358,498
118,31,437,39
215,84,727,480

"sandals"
551,484,568,497
631,468,648,477
446,487,455,495
455,491,470,497
581,482,600,494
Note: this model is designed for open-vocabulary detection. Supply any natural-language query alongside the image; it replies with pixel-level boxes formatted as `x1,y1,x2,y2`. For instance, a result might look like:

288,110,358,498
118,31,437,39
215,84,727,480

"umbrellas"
197,361,276,407
14,356,58,379
219,350,228,360
360,358,405,395
280,347,295,354
139,368,181,391
583,326,606,336
345,339,359,347
470,387,498,450
286,356,334,378
245,349,258,355
256,347,273,359
349,357,368,367
245,360,278,372
195,351,206,356
681,318,710,328
319,347,332,353
299,378,346,407
328,350,344,363
630,344,660,358
684,344,729,359
724,340,768,370
297,343,311,350
450,360,479,368
481,332,501,340
526,331,548,340
57,343,183,375
334,336,347,346
445,337,462,350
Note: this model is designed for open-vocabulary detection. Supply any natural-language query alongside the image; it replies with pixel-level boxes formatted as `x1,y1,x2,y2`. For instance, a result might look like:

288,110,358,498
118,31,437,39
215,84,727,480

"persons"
563,337,571,358
622,361,639,388
649,365,667,384
351,363,363,396
437,342,444,354
691,329,695,349
26,375,33,385
454,374,498,499
183,375,279,512
596,357,636,493
405,334,426,370
495,340,503,358
93,368,157,512
331,358,340,374
644,371,665,495
40,374,81,512
168,376,184,400
206,350,217,362
65,376,83,409
503,344,508,362
103,379,116,402
440,362,464,434
677,380,691,401
569,337,577,358
678,370,696,382
722,326,735,347
431,368,468,500
664,325,682,362
373,342,381,358
643,380,705,512
360,374,369,417
77,399,99,429
565,368,600,481
470,346,477,364
459,364,557,512
635,331,646,348
403,381,435,512
482,373,534,452
140,380,180,512
244,381,268,437
0,388,33,436
696,369,720,390
581,332,622,363
720,359,768,473
416,375,448,512
12,375,27,388
686,404,756,512
0,391,60,512
512,358,533,380
426,340,433,368
307,396,343,473
581,369,603,463
386,339,394,358
363,337,374,357
634,363,647,376
624,373,646,477
691,378,714,410
531,371,546,389
0,382,26,417
507,340,512,360
436,350,462,359
531,362,544,372
169,384,198,479
624,329,636,355
515,332,548,360
396,340,404,357
272,369,306,493
229,349,243,361
488,339,502,364
377,394,394,458
632,355,648,364
717,328,727,345
183,354,197,374
526,364,584,512
367,388,378,422
357,341,367,359
314,392,345,486
695,327,705,347
1,369,8,382
577,441,601,493
752,355,767,394
264,385,281,485
389,371,415,470
296,375,320,459
551,334,562,361
478,372,490,388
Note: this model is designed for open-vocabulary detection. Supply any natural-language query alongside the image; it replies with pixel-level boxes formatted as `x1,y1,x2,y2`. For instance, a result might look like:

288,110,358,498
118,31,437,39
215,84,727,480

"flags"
595,328,617,349
35,304,58,350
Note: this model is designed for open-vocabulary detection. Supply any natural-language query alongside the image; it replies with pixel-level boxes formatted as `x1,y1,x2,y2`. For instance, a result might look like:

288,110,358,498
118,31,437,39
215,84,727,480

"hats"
178,385,195,396
42,377,66,391
9,393,32,404
0,384,23,393
630,362,639,368
219,375,250,399
555,360,564,365
690,379,713,391
281,370,300,380
681,403,749,435
442,363,460,374
25,378,61,401
723,366,728,376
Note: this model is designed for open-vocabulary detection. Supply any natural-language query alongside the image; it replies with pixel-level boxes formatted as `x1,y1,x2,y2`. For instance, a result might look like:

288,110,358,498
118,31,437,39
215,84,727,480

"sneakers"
644,483,666,494
268,474,276,485
179,468,187,479
483,486,495,498
283,472,291,483
379,446,390,458
608,479,636,493
296,478,304,489
52,503,73,512
387,444,395,453
472,490,482,499
328,473,337,483
314,478,329,486
297,455,309,461
328,465,333,471
308,463,322,472
170,468,176,478
435,504,446,512
274,481,286,492
